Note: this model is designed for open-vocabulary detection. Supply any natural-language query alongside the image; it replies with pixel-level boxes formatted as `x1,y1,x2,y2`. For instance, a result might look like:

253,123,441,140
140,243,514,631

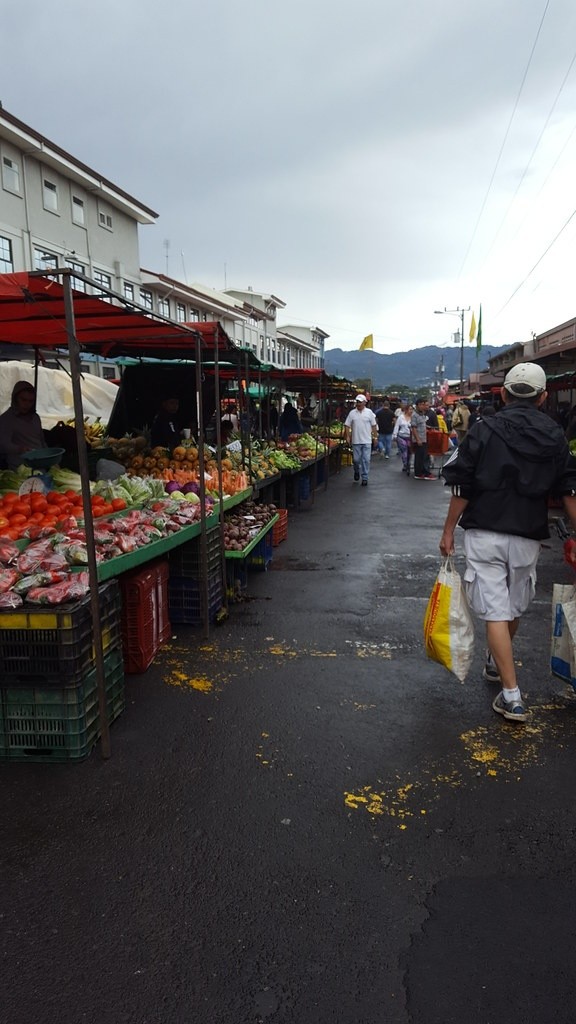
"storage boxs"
266,508,288,547
341,454,353,465
121,559,172,674
0,578,126,765
299,476,309,499
169,525,226,625
227,526,274,591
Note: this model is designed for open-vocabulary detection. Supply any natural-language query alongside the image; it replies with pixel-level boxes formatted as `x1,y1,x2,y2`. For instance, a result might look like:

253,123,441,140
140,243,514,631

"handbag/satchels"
424,554,475,681
453,407,463,427
549,583,576,688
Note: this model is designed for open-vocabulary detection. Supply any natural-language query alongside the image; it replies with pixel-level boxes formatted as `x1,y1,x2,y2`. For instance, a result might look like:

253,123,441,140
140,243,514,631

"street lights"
434,309,464,395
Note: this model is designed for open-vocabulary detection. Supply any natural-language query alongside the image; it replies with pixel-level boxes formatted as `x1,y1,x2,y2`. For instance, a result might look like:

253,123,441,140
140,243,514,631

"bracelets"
374,438,378,439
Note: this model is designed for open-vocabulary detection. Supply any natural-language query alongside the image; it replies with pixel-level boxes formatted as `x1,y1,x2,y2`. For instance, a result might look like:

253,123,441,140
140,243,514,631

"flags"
460,304,481,352
360,335,372,350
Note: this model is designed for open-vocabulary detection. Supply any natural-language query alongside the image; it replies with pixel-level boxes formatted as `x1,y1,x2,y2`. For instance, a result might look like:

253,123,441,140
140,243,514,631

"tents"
1,267,398,759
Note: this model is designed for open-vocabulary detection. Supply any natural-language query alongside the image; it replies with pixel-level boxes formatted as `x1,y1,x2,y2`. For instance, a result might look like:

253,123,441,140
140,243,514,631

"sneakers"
492,691,526,721
483,649,501,681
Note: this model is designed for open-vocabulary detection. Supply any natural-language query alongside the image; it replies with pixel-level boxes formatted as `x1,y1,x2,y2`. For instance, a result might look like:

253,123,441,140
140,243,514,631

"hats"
355,394,366,402
504,362,546,397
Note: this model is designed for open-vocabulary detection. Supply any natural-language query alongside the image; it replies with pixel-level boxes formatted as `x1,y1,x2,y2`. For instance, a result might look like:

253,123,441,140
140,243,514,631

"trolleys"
403,427,446,479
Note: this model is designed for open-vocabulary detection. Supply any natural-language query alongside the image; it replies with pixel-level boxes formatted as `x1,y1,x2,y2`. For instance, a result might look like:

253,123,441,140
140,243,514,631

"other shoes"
362,480,367,485
385,455,389,459
424,474,438,480
414,474,425,480
354,472,359,480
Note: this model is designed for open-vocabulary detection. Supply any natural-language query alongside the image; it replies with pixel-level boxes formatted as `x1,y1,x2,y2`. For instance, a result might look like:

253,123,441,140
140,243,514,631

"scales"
18,447,66,499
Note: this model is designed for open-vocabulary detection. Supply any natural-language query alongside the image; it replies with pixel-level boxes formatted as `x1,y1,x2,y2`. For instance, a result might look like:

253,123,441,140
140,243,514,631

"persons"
440,363,576,722
213,395,304,444
302,394,576,485
151,392,186,451
0,380,43,468
